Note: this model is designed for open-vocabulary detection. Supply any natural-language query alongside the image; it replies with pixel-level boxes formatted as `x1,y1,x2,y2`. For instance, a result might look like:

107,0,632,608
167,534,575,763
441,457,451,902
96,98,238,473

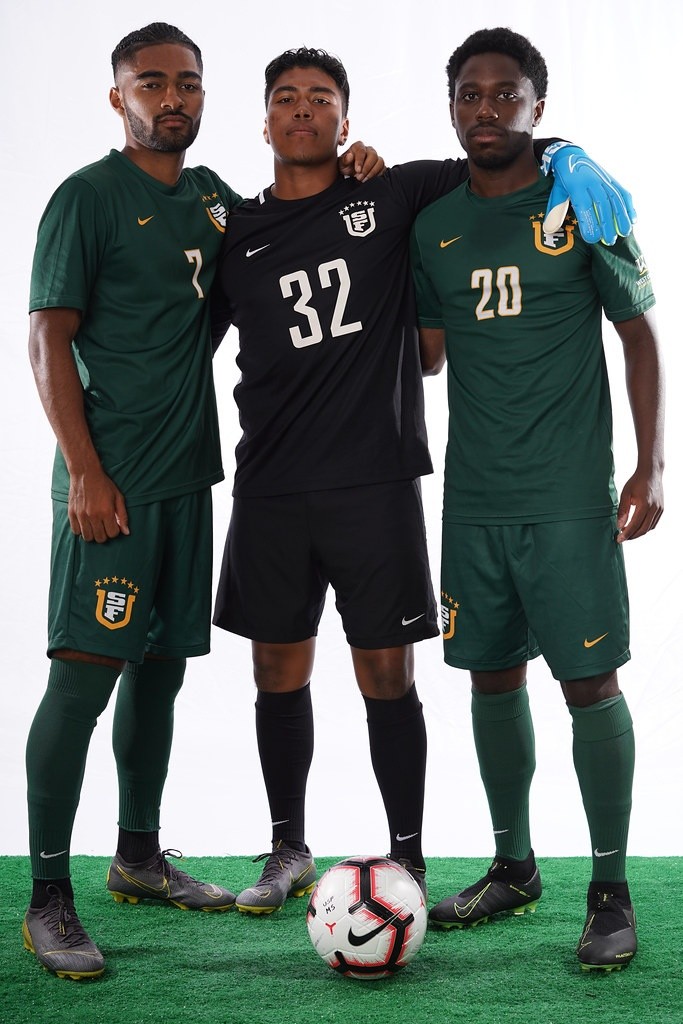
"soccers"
306,855,428,981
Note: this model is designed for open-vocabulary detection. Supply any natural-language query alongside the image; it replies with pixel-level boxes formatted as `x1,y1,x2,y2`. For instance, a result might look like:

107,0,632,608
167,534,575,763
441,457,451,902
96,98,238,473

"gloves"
539,141,636,246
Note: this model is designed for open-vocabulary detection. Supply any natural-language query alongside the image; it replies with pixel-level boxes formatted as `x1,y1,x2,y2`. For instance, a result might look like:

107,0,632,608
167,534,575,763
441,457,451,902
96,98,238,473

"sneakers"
234,839,318,915
107,848,236,913
576,890,638,972
22,895,106,980
428,857,543,929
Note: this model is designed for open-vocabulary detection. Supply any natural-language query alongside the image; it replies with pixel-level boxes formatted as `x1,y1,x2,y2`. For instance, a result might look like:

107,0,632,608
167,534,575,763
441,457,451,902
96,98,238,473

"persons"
21,20,237,981
210,47,635,913
411,26,666,972
